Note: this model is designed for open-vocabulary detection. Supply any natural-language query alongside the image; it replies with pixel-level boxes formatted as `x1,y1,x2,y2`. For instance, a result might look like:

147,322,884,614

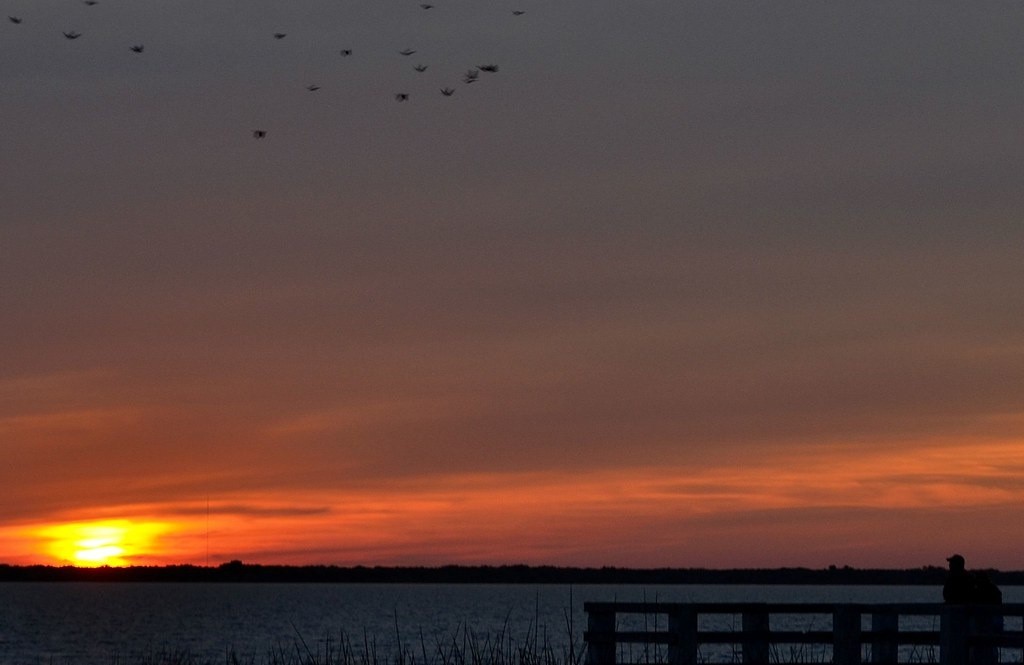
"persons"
942,556,969,601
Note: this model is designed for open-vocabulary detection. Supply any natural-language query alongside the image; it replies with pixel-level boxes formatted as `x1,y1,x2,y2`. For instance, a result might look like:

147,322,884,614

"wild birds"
274,33,287,40
395,93,409,102
413,63,429,72
8,15,22,25
400,48,415,55
439,86,457,96
130,44,145,53
63,31,82,39
512,10,525,15
420,3,434,10
341,48,353,56
253,130,268,139
461,64,500,84
306,84,321,92
84,0,99,6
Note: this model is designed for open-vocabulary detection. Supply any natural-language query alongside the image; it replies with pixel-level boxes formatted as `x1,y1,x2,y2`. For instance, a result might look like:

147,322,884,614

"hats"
946,554,963,563
